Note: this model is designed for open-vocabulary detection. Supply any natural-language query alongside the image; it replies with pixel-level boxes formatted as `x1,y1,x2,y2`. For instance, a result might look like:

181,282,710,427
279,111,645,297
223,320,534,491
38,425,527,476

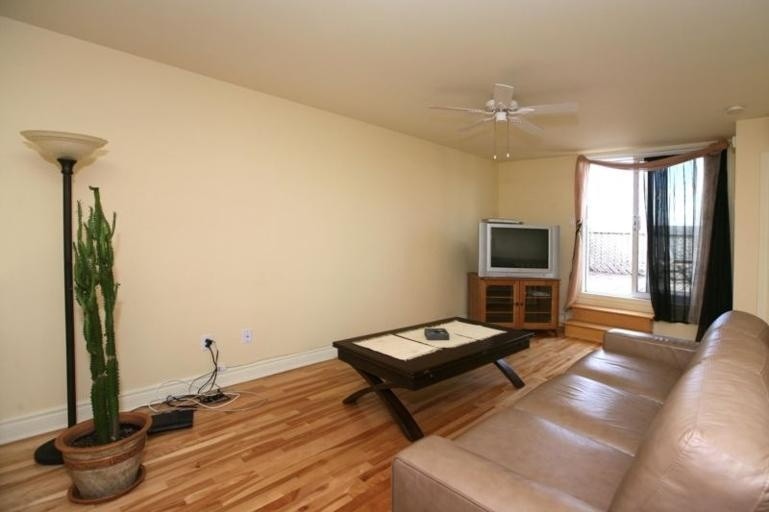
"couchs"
392,310,769,512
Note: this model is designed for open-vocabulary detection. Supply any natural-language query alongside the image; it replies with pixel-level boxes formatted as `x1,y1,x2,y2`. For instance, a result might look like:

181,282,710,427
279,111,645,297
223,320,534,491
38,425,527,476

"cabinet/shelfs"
468,273,562,337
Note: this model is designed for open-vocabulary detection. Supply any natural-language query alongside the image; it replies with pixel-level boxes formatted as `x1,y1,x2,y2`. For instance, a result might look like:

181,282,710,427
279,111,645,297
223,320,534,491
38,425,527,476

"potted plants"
54,185,154,505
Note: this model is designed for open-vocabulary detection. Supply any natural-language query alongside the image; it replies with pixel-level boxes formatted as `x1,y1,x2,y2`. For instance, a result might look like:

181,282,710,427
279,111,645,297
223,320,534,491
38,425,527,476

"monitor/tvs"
478,221,561,279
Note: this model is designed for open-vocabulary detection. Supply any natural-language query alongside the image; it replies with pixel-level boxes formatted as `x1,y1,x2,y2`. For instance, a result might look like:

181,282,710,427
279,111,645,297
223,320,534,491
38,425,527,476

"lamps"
20,130,108,465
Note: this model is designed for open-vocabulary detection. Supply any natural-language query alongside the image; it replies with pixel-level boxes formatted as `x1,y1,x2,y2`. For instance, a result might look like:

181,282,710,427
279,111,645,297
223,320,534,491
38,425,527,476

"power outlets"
201,334,212,350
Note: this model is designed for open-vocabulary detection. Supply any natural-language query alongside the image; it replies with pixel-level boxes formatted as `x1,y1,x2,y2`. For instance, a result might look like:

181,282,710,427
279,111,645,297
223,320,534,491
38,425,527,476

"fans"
427,81,579,160
